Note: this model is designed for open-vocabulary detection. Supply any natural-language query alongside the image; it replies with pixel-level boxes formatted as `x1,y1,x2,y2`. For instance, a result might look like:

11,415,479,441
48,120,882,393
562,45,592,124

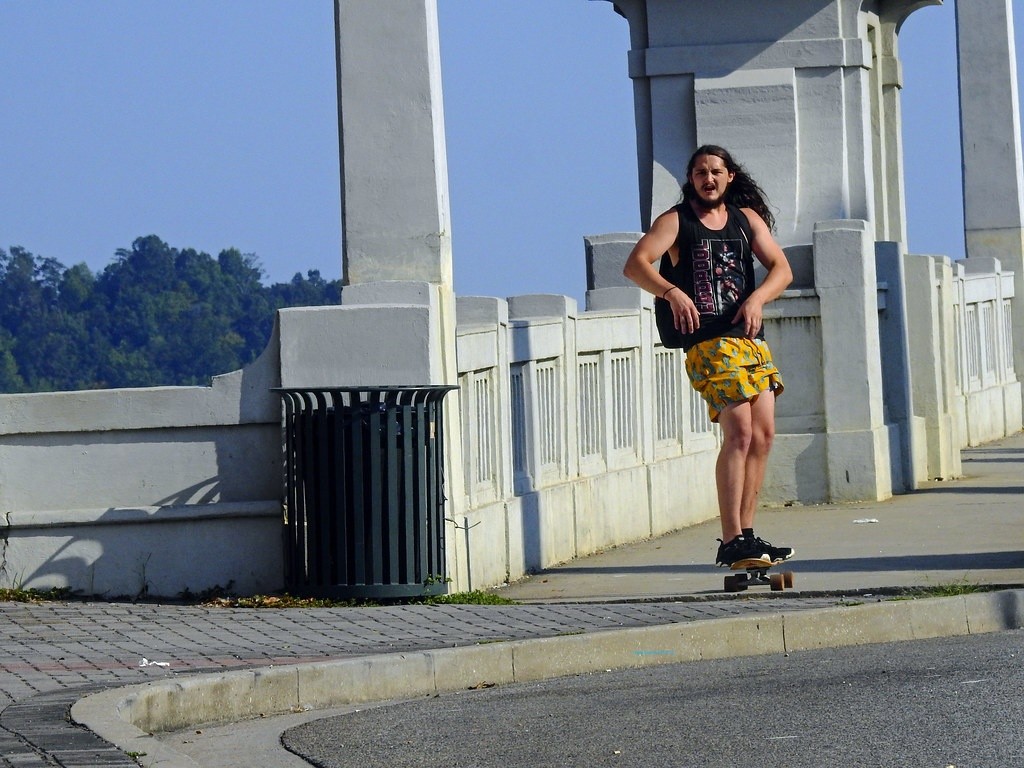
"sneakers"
716,535,783,568
748,537,797,562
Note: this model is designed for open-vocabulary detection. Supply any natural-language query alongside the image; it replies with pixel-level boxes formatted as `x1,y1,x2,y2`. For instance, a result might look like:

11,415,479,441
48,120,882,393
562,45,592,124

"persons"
624,145,795,567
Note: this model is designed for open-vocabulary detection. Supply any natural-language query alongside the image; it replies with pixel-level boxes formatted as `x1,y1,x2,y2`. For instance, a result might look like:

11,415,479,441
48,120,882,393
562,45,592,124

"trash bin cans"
269,379,460,606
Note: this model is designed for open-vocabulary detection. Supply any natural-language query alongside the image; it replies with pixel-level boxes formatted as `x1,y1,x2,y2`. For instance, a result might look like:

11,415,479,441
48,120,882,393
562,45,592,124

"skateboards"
723,558,794,593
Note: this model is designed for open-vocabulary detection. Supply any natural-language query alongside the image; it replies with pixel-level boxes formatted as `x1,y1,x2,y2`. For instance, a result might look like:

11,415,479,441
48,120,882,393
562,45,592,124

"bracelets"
663,286,677,300
